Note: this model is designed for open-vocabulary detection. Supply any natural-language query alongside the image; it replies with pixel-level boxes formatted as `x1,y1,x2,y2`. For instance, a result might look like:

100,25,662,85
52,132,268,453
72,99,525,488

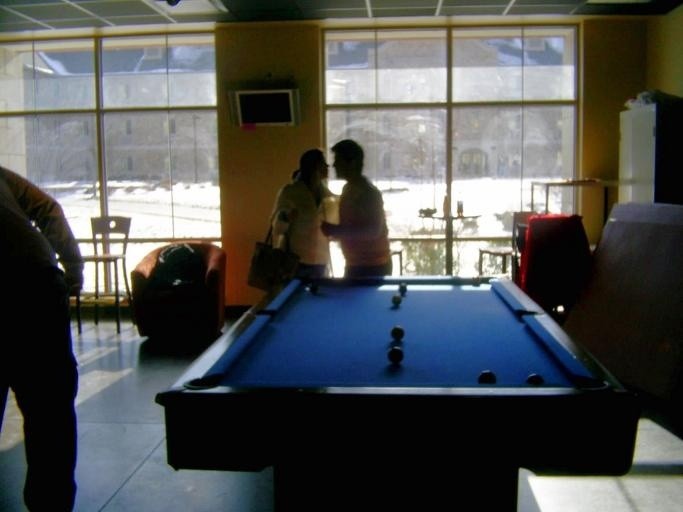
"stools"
391,248,402,274
479,247,512,275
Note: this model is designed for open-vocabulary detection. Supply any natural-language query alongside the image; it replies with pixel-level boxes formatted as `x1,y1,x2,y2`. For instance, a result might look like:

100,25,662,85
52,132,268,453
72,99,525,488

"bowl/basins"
420,210,436,217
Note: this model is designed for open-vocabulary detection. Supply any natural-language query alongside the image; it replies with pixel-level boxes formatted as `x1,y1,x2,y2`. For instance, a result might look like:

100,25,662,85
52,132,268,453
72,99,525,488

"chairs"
75,215,131,334
511,214,590,317
511,211,537,282
131,241,227,338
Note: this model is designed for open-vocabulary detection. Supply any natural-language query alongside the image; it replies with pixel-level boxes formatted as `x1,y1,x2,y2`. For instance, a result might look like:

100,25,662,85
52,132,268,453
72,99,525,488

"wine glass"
456,202,463,218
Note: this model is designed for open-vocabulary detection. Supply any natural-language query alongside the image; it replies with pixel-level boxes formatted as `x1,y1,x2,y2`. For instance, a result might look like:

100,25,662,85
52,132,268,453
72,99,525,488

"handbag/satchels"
244,238,303,295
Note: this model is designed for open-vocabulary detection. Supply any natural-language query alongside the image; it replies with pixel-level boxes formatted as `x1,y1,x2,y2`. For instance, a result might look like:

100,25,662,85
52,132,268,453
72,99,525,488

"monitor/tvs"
235,89,295,128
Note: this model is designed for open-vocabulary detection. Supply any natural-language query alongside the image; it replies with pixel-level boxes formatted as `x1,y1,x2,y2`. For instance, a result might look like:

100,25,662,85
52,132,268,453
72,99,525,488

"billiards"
391,327,404,339
478,370,495,384
392,296,402,305
526,374,543,384
398,283,407,294
309,281,320,294
388,347,403,363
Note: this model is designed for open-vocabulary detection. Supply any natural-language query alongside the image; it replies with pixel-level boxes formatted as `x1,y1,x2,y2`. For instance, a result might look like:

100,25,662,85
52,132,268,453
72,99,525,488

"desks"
155,273,640,512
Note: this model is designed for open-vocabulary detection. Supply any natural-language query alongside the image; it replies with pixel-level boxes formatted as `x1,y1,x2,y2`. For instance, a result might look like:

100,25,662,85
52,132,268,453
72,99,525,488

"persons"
271,148,341,279
318,139,394,277
0,166,86,512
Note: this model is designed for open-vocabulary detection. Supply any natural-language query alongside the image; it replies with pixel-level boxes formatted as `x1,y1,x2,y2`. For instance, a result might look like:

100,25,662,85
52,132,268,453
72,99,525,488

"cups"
516,223,527,253
